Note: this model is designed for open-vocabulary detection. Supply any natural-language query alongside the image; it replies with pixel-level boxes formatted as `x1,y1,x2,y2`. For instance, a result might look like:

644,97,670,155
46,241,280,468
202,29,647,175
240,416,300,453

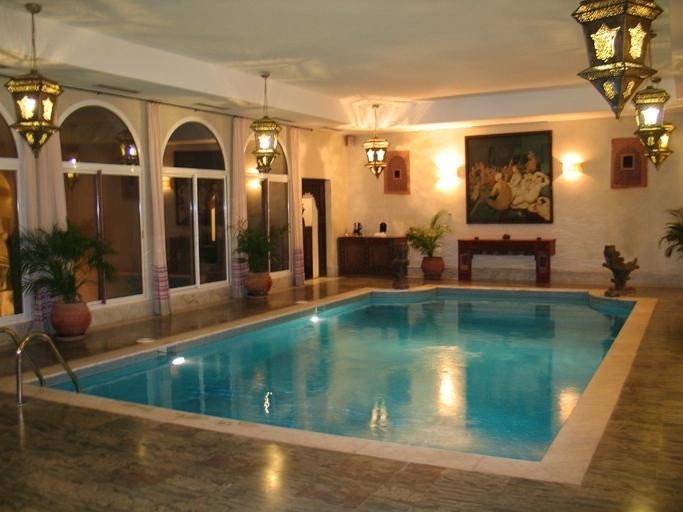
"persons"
468,147,550,220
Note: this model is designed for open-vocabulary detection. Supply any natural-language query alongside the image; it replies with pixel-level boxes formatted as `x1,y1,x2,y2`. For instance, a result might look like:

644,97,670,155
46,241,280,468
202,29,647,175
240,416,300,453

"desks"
455,239,558,283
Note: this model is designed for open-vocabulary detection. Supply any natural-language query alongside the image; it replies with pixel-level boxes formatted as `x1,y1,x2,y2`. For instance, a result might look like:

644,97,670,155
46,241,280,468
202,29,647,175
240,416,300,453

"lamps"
249,73,281,174
64,145,80,190
2,3,64,162
630,33,670,152
569,1,663,117
361,104,390,179
116,127,138,164
642,76,675,169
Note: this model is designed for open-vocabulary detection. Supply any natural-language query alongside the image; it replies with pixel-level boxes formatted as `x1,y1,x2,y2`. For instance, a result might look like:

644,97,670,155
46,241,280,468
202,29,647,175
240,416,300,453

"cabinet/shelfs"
336,233,407,281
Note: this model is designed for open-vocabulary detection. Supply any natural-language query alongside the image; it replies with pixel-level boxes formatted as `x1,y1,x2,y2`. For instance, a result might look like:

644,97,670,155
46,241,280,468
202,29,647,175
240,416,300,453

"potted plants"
228,215,291,302
403,208,458,283
2,220,119,344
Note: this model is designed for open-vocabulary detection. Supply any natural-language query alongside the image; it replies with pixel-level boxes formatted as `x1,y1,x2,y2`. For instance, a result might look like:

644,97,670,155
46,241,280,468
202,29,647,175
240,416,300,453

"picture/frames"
461,130,553,224
